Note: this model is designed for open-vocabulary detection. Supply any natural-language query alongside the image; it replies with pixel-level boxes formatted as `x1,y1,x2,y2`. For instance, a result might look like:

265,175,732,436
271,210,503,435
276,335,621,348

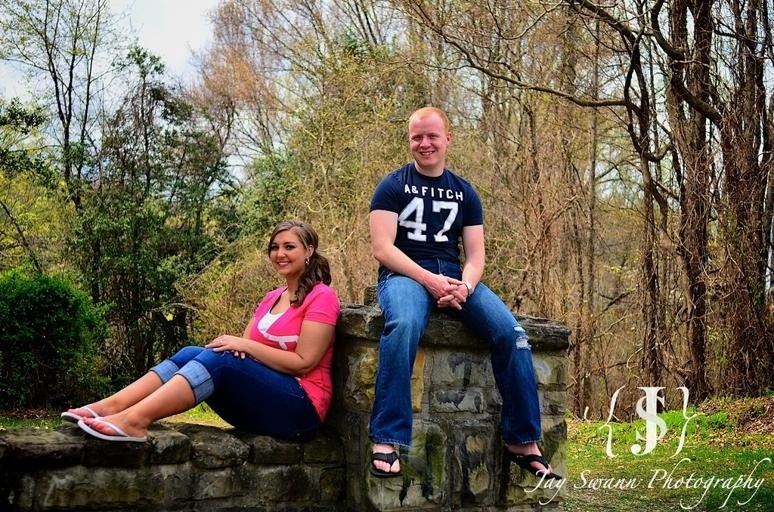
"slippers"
369,451,401,478
503,447,562,481
60,406,147,442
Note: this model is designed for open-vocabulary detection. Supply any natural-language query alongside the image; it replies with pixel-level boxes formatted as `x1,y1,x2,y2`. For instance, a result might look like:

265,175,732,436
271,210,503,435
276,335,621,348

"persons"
365,108,563,480
57,219,342,441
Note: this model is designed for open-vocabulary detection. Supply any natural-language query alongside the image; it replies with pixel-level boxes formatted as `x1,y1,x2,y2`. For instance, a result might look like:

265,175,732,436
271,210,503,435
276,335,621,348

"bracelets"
460,280,474,297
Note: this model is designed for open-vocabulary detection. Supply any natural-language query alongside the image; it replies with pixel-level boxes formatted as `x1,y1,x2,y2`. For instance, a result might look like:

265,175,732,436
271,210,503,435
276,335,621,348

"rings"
219,339,224,346
